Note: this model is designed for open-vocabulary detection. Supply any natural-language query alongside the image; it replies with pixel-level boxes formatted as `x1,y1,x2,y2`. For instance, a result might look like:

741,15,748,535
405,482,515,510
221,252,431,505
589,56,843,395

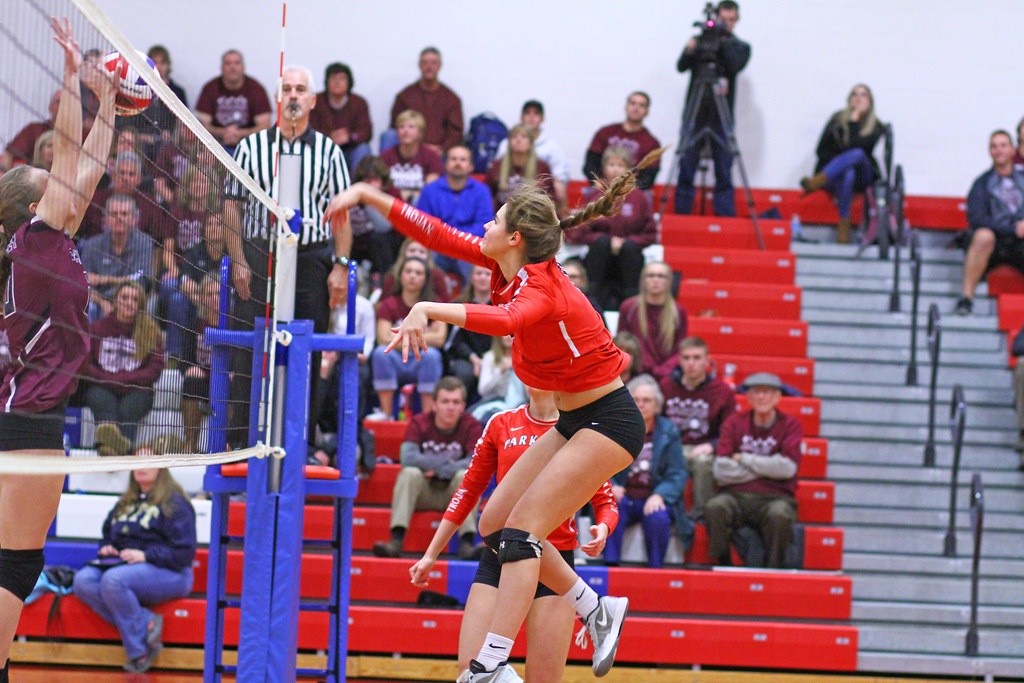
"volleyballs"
97,49,160,117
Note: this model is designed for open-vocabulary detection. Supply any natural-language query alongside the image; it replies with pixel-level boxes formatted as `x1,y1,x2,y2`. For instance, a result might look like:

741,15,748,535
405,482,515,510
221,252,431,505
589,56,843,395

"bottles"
791,213,801,240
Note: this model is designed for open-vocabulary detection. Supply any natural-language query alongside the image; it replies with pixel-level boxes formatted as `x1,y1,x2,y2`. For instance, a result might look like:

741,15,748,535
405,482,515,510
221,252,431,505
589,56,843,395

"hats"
743,373,781,388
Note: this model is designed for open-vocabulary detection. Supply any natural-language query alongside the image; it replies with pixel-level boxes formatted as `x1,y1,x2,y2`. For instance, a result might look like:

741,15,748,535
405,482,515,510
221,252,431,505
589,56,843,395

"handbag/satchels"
24,566,78,623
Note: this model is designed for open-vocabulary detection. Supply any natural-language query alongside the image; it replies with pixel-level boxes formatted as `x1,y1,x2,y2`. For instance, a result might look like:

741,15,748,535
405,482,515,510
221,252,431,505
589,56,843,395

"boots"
800,172,827,197
226,404,233,452
180,399,200,454
837,216,850,243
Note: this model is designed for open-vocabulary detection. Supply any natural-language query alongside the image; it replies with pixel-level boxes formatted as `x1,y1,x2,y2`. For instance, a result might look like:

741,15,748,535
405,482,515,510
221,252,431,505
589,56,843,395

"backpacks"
469,113,508,172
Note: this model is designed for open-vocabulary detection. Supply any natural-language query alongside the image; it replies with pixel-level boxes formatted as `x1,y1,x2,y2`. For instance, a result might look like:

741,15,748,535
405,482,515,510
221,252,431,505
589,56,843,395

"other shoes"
125,648,158,672
147,615,162,648
366,412,394,421
372,540,401,558
955,297,973,315
95,423,132,452
167,360,180,369
458,542,484,561
98,446,119,473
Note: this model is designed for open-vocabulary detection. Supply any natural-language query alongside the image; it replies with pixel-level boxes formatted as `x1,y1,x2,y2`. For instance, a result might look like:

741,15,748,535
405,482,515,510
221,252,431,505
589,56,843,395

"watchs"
331,254,352,270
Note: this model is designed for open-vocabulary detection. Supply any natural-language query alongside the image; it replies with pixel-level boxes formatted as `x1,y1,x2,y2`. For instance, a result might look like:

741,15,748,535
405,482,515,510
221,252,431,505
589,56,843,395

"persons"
618,261,687,384
800,84,886,243
0,15,800,683
372,375,488,559
674,0,752,216
945,112,1024,361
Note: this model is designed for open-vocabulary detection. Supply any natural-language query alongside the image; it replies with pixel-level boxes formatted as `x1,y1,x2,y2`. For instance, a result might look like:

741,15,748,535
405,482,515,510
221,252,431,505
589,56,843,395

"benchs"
0,165,1024,683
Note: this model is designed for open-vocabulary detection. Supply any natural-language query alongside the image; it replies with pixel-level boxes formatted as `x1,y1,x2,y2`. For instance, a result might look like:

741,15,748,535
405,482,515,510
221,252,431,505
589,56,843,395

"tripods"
661,60,765,250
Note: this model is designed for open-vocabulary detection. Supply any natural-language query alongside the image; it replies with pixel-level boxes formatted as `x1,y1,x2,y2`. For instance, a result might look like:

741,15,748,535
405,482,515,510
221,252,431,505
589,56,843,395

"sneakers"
456,659,523,683
574,596,629,677
